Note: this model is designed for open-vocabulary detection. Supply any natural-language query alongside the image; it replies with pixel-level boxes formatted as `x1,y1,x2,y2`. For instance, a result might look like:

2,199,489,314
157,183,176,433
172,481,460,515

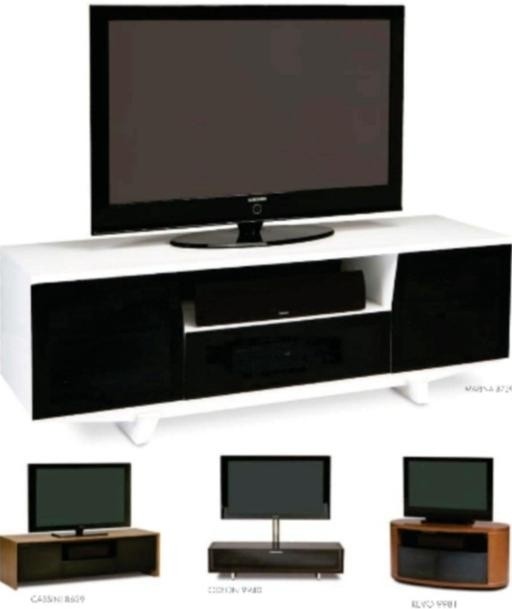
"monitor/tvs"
88,5,404,249
28,463,130,538
403,456,493,525
221,456,331,520
